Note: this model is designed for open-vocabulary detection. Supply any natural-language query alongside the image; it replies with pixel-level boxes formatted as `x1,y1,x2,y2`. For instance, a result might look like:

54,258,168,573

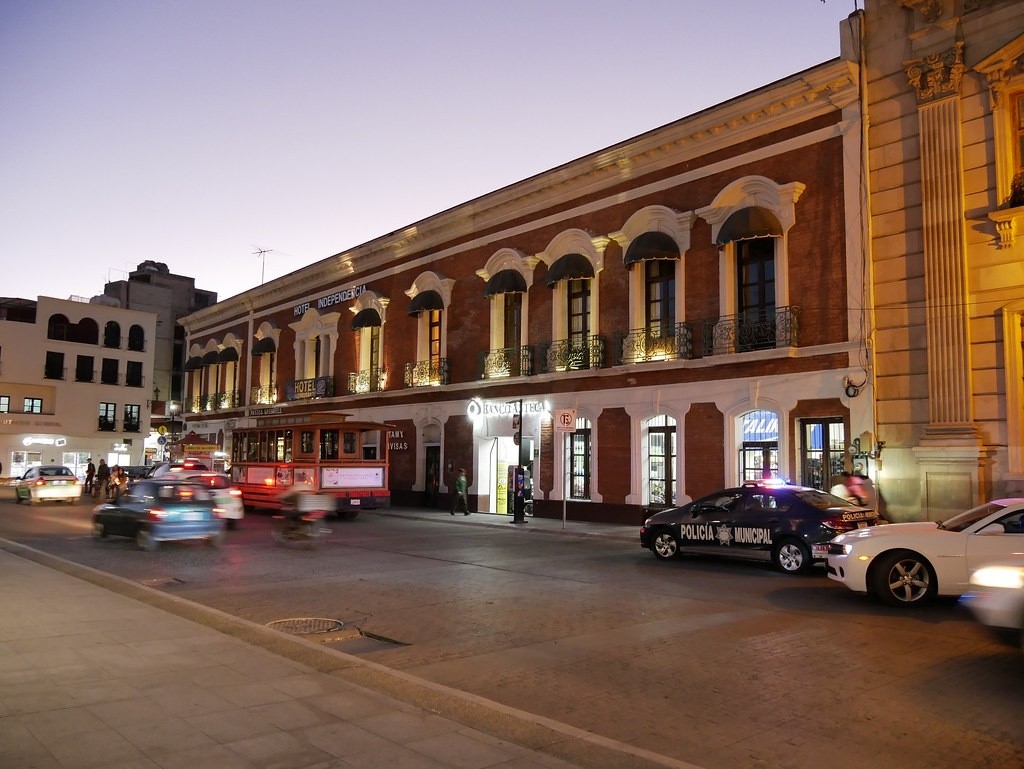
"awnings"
624,232,681,271
183,348,238,372
484,270,527,301
351,308,381,332
408,291,444,318
546,254,595,290
716,207,783,253
251,338,275,356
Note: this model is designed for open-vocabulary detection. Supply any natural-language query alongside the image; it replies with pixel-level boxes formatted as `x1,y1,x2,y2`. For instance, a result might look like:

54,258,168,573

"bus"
230,411,396,521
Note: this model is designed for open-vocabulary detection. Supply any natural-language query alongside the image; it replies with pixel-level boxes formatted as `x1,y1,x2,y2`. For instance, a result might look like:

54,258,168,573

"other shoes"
450,511,455,515
84,490,88,493
465,511,470,516
88,491,92,494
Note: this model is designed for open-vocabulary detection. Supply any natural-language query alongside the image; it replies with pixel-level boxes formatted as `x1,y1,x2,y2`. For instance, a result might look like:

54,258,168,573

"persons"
83,458,127,499
840,472,879,527
450,468,471,517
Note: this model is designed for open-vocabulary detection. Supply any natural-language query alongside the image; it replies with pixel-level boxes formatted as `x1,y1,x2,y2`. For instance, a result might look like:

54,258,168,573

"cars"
960,552,1024,647
640,478,880,576
15,465,82,506
90,465,153,498
158,470,245,530
825,498,1024,611
145,462,209,478
90,478,225,552
